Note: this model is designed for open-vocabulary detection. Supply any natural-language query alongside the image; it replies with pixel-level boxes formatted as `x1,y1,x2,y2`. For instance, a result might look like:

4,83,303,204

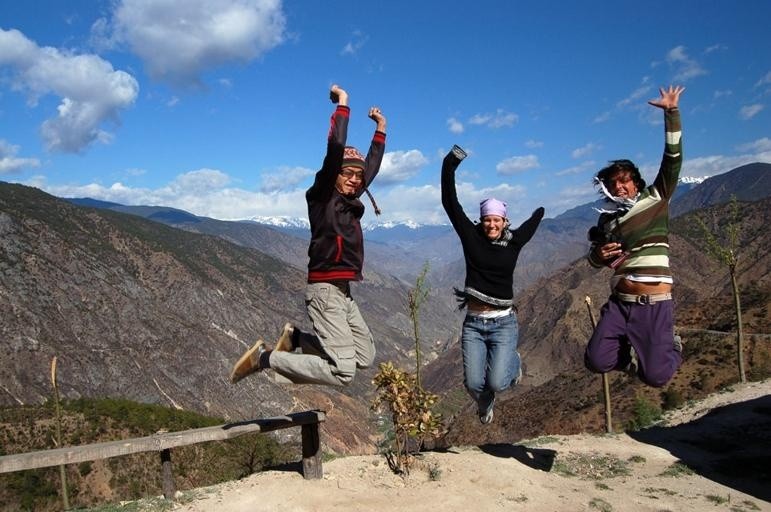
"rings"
608,252,611,257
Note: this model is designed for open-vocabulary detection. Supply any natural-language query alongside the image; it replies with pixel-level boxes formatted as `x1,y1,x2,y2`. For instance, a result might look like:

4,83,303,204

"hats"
480,199,508,219
587,228,632,268
341,145,365,170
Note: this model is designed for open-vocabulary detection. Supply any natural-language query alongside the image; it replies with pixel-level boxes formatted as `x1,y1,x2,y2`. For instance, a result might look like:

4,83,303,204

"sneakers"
510,351,524,387
627,347,638,377
478,389,495,425
673,336,683,354
276,322,297,353
229,338,273,384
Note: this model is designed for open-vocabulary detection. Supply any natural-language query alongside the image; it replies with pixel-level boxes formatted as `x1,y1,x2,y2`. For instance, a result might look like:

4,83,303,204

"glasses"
337,169,363,180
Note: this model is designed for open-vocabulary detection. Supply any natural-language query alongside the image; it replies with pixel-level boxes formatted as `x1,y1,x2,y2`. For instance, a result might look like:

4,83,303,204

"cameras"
589,229,622,250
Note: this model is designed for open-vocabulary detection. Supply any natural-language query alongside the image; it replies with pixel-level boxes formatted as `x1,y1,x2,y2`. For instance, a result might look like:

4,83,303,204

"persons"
439,144,546,428
229,84,389,387
583,82,690,388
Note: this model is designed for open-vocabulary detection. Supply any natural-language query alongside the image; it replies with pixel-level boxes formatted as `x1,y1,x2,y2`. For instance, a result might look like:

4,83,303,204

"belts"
615,291,674,306
